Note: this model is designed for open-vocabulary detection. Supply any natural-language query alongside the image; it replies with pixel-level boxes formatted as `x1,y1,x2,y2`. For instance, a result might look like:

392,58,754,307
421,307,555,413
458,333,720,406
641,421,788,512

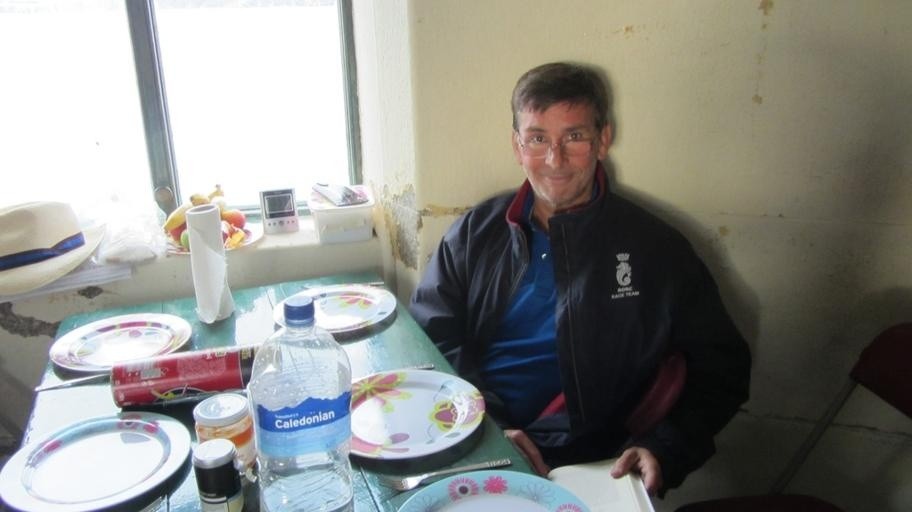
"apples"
170,208,246,247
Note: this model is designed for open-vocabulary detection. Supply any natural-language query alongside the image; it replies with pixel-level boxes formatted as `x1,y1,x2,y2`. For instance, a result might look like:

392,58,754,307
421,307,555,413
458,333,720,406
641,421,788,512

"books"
545,455,655,512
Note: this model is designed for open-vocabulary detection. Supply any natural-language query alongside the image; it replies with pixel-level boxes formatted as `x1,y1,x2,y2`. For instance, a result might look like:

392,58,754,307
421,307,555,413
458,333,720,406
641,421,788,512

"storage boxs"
308,185,373,243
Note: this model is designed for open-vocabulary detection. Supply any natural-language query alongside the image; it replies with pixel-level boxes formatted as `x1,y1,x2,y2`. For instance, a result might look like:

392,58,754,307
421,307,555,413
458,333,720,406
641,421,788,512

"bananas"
163,180,222,231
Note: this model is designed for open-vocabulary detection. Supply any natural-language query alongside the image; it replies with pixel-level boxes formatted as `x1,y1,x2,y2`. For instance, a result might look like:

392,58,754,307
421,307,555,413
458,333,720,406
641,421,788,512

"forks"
373,459,518,491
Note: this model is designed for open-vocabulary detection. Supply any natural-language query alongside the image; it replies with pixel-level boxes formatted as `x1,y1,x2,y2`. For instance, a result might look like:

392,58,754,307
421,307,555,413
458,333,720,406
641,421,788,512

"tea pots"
89,163,174,265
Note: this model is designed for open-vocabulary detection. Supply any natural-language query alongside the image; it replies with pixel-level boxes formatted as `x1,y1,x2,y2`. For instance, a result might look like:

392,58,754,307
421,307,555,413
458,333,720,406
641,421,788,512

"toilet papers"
185,203,237,326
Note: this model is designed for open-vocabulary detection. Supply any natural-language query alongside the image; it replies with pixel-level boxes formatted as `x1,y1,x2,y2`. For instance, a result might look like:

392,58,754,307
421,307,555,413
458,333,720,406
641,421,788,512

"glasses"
516,130,601,157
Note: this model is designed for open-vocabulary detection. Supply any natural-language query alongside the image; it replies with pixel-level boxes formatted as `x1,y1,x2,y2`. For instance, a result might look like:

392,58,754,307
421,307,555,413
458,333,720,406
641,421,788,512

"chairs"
676,319,912,512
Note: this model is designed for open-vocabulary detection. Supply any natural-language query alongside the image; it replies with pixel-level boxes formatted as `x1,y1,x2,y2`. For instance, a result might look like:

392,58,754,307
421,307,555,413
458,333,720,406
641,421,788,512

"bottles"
247,295,354,511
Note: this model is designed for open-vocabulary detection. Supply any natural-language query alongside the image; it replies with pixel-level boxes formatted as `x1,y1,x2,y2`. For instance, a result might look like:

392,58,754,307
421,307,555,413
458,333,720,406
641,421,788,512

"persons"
407,63,751,497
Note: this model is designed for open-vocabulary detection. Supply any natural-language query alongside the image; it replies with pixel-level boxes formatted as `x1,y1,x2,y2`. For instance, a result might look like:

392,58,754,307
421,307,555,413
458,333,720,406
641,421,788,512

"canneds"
191,437,245,511
192,394,256,475
108,345,259,408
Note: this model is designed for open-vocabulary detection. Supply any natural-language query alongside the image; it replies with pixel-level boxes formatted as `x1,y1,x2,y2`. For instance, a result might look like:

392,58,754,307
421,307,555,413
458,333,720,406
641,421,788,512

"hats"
0,201,107,297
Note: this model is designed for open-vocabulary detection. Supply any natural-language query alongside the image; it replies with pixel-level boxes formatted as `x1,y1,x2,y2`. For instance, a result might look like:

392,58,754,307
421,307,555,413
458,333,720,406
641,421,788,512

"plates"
48,313,197,374
350,368,492,463
395,469,595,511
268,280,400,334
1,408,192,512
163,222,270,254
